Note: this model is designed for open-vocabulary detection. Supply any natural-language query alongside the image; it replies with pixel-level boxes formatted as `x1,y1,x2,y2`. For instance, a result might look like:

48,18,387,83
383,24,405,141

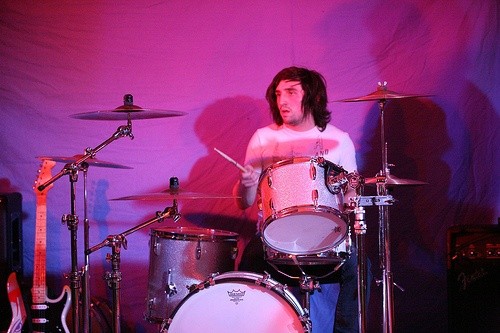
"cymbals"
106,188,243,200
70,105,189,121
332,91,433,101
364,176,436,187
35,153,134,170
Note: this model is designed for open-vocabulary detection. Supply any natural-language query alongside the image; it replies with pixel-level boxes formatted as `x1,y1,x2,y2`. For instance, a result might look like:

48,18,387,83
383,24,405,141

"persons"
232,66,359,333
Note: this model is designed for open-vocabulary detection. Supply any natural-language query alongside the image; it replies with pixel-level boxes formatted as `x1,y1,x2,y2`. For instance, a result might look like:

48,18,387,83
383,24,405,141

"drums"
257,156,351,255
262,220,352,265
141,225,240,325
160,271,312,333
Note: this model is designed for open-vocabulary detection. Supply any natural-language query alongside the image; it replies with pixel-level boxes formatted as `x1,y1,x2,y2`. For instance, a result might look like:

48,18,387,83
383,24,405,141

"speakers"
0,192,25,333
446,259,500,333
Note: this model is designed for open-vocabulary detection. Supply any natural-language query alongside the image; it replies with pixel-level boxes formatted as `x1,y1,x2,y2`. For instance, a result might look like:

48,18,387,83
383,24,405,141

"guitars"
7,160,71,333
